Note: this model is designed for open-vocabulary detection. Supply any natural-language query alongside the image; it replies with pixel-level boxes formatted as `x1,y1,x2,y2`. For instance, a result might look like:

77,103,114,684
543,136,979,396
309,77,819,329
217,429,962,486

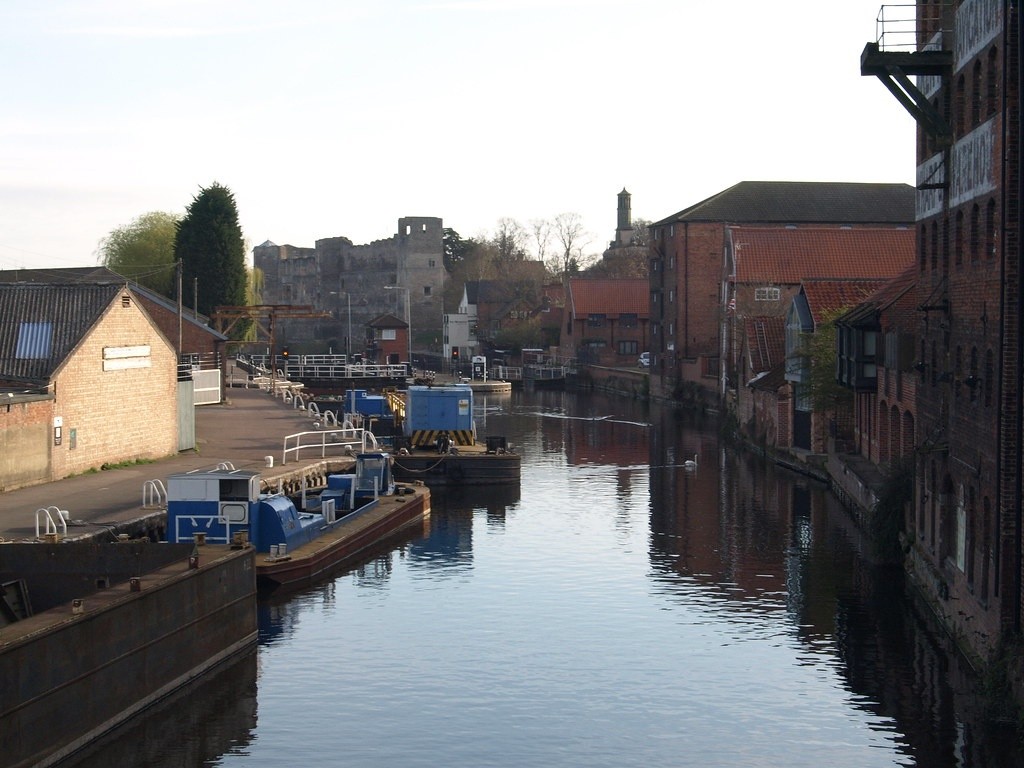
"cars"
637,352,650,368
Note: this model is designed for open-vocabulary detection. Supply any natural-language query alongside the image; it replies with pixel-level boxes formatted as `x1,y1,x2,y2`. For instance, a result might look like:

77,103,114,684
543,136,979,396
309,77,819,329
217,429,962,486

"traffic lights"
452,346,458,359
283,347,289,359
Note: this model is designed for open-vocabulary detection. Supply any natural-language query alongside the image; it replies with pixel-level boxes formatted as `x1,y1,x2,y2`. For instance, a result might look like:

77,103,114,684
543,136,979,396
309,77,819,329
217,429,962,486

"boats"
341,383,521,485
152,445,431,595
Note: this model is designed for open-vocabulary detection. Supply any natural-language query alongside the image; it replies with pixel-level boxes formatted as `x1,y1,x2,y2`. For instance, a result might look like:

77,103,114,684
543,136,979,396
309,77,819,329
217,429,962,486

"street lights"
329,292,352,362
384,286,411,366
